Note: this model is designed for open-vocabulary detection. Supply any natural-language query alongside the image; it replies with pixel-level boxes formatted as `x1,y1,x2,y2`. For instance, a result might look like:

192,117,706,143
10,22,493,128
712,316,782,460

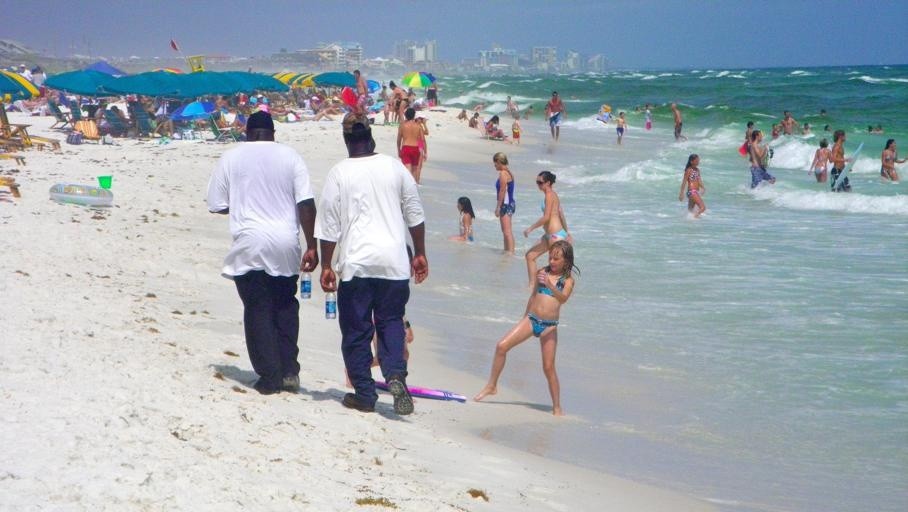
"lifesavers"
50,182,115,205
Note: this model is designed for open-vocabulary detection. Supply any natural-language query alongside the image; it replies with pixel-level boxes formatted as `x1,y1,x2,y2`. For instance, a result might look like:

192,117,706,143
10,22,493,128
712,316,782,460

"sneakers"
386,375,415,413
283,375,300,391
342,392,377,412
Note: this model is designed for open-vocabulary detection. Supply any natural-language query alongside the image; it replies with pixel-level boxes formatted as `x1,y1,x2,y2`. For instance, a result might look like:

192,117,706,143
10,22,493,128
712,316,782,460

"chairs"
46,95,242,144
1,103,32,165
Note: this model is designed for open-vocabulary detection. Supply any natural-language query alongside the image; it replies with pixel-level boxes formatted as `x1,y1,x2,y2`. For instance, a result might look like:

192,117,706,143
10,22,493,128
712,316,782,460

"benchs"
27,134,61,152
0,175,21,198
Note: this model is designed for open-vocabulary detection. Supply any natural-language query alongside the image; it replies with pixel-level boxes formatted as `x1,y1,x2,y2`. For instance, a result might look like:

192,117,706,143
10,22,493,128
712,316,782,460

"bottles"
325,289,337,320
301,271,312,300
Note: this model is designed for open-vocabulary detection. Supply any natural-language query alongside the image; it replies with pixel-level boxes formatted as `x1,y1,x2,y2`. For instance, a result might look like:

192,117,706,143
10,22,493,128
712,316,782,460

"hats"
246,109,273,129
342,112,370,135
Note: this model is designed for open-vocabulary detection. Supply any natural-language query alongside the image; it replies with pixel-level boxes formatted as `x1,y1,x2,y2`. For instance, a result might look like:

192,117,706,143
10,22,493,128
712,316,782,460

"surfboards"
833,143,864,190
